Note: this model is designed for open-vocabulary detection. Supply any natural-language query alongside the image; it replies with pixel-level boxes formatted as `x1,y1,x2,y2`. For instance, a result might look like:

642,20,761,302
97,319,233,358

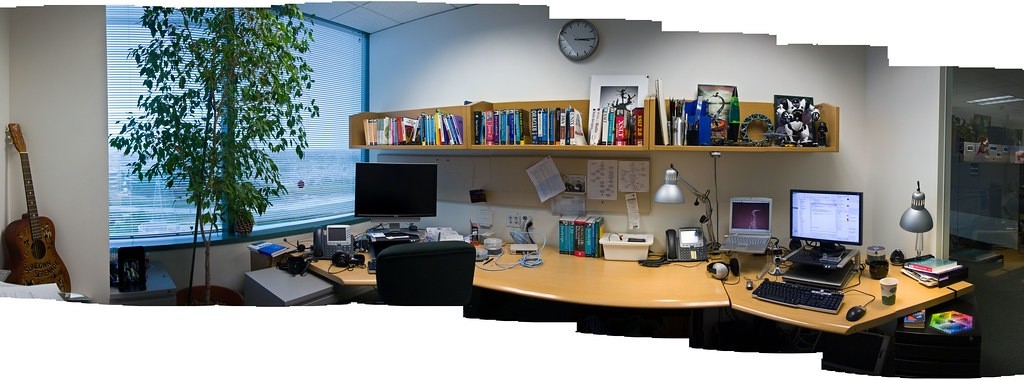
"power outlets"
506,212,535,229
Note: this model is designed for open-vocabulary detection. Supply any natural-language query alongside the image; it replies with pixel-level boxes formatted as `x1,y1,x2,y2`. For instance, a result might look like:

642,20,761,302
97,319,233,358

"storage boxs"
599,234,653,261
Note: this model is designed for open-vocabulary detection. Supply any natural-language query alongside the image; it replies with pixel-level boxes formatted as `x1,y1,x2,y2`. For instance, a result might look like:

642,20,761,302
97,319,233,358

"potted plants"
108,5,320,307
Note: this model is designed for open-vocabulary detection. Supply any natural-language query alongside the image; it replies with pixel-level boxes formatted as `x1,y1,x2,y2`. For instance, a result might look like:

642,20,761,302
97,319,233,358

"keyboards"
367,261,376,274
752,280,845,315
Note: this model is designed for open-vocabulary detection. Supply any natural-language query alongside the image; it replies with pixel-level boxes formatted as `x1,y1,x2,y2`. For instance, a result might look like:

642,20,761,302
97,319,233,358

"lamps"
711,151,721,254
654,163,720,254
900,181,934,264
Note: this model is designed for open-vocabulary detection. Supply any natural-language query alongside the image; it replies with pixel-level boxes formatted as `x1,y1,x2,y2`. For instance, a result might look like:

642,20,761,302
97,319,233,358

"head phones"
707,257,742,280
279,257,305,275
332,251,365,267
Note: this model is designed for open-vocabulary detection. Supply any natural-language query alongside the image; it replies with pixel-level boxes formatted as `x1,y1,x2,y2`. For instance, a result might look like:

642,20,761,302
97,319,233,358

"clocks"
558,20,599,61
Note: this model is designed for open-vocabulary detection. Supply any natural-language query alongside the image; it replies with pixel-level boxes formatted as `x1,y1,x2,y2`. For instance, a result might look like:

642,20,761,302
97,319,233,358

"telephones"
310,224,354,260
665,227,708,262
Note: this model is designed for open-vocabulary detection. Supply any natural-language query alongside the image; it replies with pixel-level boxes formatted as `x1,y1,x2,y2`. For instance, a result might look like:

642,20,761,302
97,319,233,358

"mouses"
846,305,866,321
746,281,753,290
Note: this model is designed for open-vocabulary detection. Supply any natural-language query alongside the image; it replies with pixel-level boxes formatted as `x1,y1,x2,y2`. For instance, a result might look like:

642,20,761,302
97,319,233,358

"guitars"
5,122,72,294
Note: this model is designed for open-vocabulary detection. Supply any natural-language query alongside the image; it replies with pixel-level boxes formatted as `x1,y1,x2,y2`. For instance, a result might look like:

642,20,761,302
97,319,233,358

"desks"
288,242,976,378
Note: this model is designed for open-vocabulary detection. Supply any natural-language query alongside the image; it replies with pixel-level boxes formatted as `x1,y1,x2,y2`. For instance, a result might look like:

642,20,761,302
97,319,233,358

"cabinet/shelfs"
244,266,338,307
250,250,287,271
110,261,177,306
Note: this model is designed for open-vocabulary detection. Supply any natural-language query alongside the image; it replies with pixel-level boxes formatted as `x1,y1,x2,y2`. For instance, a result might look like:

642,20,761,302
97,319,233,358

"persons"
749,217,756,228
565,181,582,192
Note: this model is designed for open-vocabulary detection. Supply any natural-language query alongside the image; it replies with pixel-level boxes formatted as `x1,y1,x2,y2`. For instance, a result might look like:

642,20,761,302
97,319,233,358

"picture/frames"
118,246,147,292
588,73,648,145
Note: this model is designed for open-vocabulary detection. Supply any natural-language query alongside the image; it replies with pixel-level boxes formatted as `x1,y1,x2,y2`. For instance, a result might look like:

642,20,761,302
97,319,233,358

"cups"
870,260,889,279
880,278,898,306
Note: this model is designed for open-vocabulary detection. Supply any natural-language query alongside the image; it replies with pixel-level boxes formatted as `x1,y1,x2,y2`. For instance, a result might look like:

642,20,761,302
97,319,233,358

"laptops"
781,264,854,289
719,197,772,254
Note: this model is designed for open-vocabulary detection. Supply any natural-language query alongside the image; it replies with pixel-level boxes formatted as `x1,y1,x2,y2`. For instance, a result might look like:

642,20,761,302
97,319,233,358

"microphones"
301,260,310,275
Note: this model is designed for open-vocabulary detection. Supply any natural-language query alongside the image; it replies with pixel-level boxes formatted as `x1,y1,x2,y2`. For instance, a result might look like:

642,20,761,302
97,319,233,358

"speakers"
889,248,906,266
810,331,892,376
789,239,804,251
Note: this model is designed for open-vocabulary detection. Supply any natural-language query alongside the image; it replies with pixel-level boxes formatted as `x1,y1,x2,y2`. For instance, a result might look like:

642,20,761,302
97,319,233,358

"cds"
867,246,885,251
475,244,501,261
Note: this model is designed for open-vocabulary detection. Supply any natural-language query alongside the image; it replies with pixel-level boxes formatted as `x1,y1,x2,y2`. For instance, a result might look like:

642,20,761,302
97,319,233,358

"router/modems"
510,244,539,255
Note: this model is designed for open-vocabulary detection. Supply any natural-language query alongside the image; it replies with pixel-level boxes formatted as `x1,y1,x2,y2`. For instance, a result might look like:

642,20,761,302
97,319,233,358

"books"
908,256,957,273
474,105,643,145
363,109,463,146
559,215,604,258
247,241,288,258
904,309,925,328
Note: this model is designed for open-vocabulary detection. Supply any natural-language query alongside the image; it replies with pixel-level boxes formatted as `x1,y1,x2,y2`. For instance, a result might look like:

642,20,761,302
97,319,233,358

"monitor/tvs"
354,162,438,235
790,189,864,257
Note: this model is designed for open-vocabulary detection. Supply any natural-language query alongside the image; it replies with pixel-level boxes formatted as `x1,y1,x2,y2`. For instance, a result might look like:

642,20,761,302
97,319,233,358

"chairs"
368,240,476,319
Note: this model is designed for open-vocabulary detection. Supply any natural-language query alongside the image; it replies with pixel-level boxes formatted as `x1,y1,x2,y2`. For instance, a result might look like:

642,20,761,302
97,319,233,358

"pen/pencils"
483,258,493,265
